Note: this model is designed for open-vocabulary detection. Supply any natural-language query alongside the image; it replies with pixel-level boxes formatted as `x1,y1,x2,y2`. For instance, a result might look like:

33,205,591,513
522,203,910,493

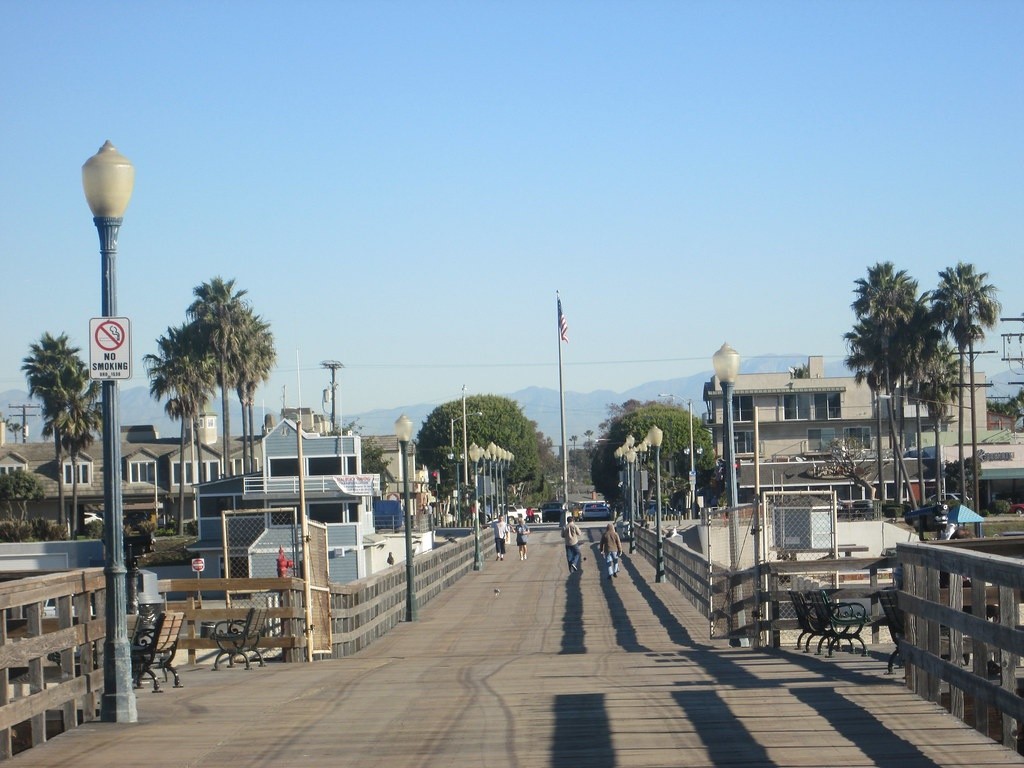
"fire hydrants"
276,544,295,662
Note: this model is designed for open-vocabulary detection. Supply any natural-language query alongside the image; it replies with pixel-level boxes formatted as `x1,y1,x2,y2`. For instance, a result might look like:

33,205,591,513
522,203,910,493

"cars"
926,492,970,505
53,512,102,537
497,504,519,525
1005,497,1024,514
852,499,874,509
515,501,614,523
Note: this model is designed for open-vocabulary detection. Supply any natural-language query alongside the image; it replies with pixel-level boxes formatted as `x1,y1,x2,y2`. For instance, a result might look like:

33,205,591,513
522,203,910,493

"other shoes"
521,557,523,561
501,558,503,561
524,554,527,559
613,573,617,577
607,575,612,579
496,556,500,561
570,571,573,573
572,564,577,570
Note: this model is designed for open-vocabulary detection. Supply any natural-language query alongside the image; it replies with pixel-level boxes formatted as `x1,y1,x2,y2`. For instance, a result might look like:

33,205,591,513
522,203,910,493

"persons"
599,523,623,578
937,524,977,588
561,516,582,573
494,507,534,560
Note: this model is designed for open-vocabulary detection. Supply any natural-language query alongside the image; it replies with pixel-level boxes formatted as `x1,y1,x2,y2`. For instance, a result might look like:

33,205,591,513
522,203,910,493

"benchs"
46,614,141,673
130,611,185,693
209,608,268,672
879,592,905,675
787,591,843,655
808,590,869,658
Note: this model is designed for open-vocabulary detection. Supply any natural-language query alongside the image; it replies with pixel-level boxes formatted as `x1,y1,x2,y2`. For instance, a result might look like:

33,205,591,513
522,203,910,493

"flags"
558,297,568,343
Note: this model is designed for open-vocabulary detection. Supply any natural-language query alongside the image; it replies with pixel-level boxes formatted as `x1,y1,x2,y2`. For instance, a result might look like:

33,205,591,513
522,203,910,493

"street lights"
648,424,666,583
469,441,515,570
711,340,750,648
81,140,136,724
613,434,652,555
450,412,482,448
462,383,468,486
447,452,464,528
394,414,418,622
657,393,696,519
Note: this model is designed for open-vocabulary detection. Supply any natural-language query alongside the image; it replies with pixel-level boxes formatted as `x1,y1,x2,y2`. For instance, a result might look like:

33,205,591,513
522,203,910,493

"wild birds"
494,585,501,597
386,551,395,570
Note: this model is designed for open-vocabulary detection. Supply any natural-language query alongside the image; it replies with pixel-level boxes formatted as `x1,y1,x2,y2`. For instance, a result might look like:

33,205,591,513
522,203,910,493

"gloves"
601,552,604,556
618,552,622,556
